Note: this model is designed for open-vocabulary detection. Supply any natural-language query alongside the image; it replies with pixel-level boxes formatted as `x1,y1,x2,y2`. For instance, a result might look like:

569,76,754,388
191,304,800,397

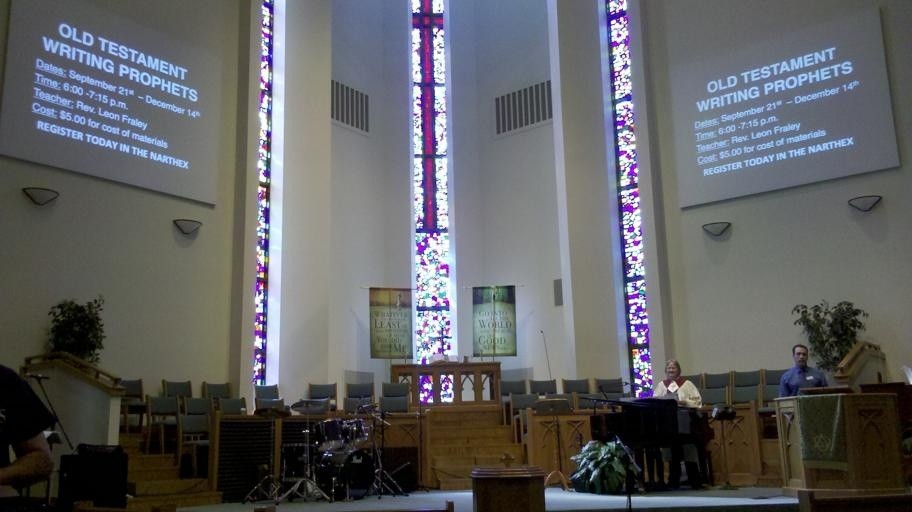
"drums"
314,447,376,501
343,419,369,448
281,444,313,481
311,417,347,453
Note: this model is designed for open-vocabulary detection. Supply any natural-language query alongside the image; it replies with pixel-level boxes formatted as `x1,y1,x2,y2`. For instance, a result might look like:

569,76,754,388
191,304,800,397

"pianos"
579,396,709,490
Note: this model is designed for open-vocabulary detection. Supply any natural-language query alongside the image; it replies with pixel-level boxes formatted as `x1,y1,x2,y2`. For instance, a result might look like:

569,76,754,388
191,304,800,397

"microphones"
25,373,49,379
598,385,608,399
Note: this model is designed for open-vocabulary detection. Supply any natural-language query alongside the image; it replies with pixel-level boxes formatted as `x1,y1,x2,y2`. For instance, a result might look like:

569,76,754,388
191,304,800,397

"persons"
653,358,707,491
0,362,56,486
778,344,828,397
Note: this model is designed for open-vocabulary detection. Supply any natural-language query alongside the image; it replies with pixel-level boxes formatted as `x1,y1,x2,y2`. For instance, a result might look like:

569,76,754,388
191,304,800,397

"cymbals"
291,401,335,415
254,407,292,419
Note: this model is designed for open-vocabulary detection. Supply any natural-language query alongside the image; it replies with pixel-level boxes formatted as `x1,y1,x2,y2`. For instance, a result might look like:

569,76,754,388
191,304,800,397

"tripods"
241,416,293,506
375,419,410,499
275,414,332,505
534,398,572,492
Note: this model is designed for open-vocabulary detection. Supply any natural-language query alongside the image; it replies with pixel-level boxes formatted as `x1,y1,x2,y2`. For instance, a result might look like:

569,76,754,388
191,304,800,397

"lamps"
848,195,881,212
24,185,57,207
174,218,203,234
702,221,730,236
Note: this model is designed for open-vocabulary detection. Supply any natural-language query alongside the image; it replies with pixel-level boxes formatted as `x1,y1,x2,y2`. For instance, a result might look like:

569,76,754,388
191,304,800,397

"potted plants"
568,439,602,492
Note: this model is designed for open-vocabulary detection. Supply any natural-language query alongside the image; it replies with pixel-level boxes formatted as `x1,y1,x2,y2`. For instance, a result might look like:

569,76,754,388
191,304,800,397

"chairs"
763,369,789,400
201,381,232,408
309,381,337,412
509,393,539,442
595,378,623,393
344,382,373,413
163,379,193,421
254,384,284,413
380,382,409,413
702,372,731,405
145,393,182,460
730,371,763,406
503,380,526,425
218,397,247,414
683,374,704,396
561,378,588,394
119,379,148,427
178,396,214,472
530,379,557,396
